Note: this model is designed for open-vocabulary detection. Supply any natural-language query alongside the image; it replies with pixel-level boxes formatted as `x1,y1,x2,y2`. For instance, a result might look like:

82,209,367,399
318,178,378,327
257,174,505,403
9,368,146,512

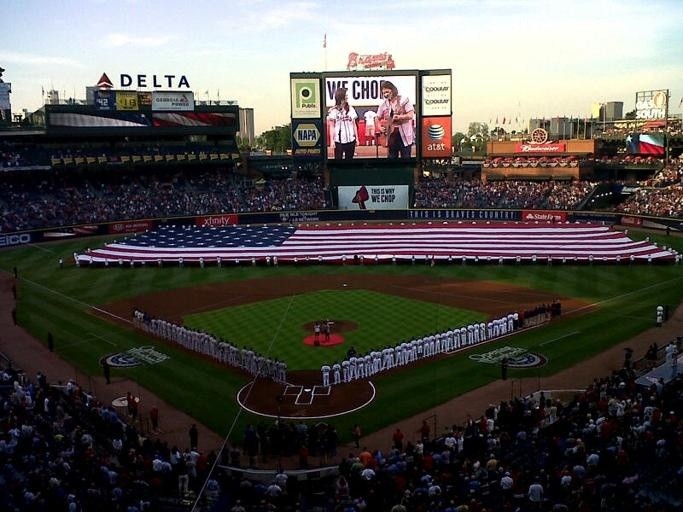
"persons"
57,248,183,266
132,308,288,386
309,334,682,512
326,87,361,160
320,300,562,386
1,357,315,511
313,321,322,339
294,253,652,266
13,265,19,280
655,302,671,326
1,148,682,234
198,255,278,267
375,81,416,160
668,248,681,264
361,107,379,146
48,331,55,352
13,283,16,299
11,307,17,328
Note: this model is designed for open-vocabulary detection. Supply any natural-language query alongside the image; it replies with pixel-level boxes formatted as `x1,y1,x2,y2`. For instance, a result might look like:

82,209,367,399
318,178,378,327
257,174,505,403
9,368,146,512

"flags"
76,222,676,263
